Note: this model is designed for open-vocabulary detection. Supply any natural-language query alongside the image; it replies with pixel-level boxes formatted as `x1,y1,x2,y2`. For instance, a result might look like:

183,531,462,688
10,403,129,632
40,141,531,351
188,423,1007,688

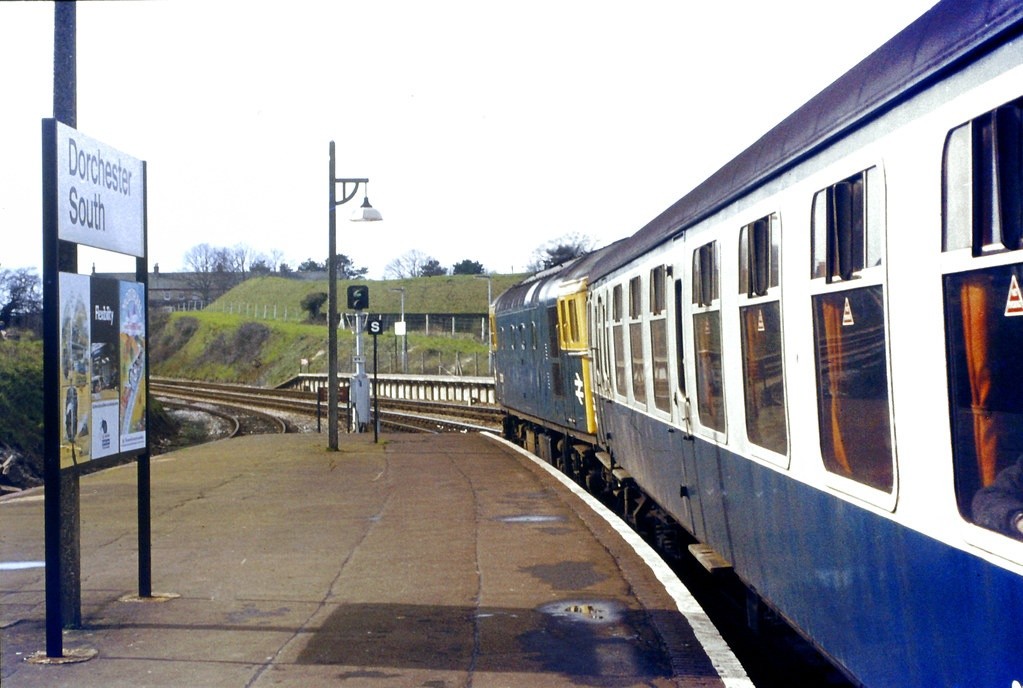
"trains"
489,0,1023,688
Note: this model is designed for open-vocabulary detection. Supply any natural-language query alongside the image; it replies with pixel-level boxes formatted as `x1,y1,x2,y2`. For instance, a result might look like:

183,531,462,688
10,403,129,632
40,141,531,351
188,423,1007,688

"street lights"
473,276,493,375
390,288,406,371
326,141,383,450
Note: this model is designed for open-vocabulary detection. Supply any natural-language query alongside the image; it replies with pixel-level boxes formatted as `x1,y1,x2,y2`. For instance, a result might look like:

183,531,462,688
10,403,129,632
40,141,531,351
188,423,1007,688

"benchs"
824,399,894,484
955,408,1023,516
